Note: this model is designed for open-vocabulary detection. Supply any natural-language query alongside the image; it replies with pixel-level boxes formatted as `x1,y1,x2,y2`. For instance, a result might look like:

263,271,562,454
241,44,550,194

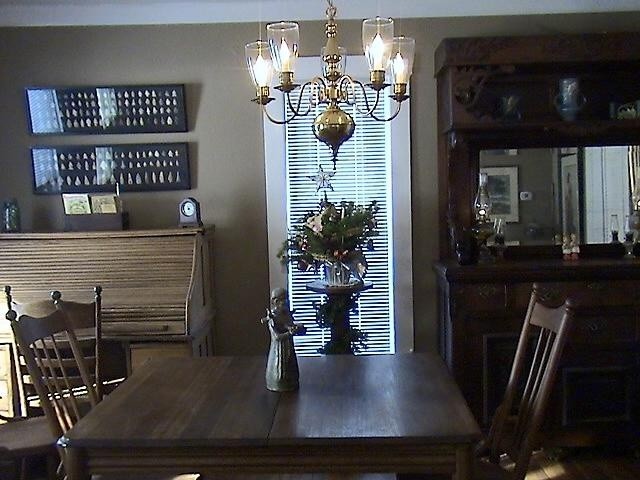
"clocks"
176,198,201,226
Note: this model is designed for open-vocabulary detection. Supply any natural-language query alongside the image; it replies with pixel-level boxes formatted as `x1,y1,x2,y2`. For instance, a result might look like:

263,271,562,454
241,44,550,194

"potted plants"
276,193,383,355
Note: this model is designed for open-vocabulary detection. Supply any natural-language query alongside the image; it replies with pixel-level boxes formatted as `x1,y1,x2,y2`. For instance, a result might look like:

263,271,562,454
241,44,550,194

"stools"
1,414,65,480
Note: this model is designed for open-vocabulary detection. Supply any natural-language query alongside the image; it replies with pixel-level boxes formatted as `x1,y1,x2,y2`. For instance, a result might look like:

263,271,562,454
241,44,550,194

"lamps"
242,0,419,178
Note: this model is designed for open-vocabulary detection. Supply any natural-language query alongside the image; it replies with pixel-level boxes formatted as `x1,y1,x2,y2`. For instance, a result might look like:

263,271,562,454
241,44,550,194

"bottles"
555,79,588,123
3,198,21,233
494,217,506,258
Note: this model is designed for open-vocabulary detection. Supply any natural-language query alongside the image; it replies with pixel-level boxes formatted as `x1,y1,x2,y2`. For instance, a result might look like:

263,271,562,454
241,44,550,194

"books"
60,193,122,214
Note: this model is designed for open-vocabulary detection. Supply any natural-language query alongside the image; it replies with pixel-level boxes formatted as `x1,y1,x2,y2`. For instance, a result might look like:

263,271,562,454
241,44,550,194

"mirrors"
476,144,639,249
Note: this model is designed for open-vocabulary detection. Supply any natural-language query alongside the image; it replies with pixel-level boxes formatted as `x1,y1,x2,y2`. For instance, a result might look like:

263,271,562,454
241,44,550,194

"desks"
0,222,218,423
58,354,483,480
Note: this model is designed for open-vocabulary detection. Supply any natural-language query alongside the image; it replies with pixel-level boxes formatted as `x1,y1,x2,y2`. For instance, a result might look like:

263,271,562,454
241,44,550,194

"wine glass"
622,215,637,260
610,215,621,246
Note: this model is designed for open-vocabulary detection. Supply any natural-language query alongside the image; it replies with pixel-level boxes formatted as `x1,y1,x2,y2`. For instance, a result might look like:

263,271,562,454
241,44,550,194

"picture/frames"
479,164,521,225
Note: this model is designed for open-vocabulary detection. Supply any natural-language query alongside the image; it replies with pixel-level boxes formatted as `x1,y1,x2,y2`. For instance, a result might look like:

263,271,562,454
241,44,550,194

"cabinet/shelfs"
435,264,639,430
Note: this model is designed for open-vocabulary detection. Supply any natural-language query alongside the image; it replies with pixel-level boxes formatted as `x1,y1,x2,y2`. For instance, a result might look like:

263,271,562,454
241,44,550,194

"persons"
262,288,307,392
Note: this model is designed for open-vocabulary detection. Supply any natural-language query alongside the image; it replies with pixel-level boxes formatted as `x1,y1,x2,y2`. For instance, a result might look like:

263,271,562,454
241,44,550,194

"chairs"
393,283,577,478
0,280,155,480
